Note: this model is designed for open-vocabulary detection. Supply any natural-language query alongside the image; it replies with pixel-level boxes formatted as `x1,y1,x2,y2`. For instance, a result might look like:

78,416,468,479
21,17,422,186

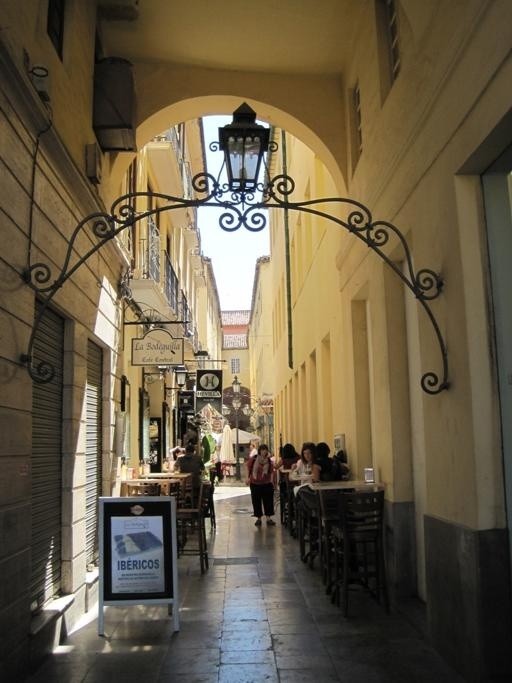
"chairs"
274,467,387,604
122,471,219,574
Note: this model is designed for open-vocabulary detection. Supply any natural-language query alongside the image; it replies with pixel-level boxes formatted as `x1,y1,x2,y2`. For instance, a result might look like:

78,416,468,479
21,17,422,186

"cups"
304,463,312,475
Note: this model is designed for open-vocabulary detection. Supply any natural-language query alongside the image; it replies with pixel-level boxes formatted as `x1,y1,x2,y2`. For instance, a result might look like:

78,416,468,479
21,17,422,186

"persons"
169,442,353,526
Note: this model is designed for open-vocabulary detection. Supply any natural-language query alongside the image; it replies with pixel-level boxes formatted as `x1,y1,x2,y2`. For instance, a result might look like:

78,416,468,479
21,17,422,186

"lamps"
216,98,273,193
161,369,188,392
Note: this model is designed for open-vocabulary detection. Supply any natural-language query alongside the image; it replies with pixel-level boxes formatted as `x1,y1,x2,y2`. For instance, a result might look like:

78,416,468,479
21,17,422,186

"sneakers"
267,519,275,525
255,520,261,525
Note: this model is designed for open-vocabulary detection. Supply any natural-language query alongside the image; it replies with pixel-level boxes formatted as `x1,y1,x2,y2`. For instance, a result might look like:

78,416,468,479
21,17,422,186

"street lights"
221,375,257,478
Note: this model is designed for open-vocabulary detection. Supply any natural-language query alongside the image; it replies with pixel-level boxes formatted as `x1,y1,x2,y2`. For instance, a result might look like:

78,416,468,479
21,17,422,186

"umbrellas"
219,423,238,483
